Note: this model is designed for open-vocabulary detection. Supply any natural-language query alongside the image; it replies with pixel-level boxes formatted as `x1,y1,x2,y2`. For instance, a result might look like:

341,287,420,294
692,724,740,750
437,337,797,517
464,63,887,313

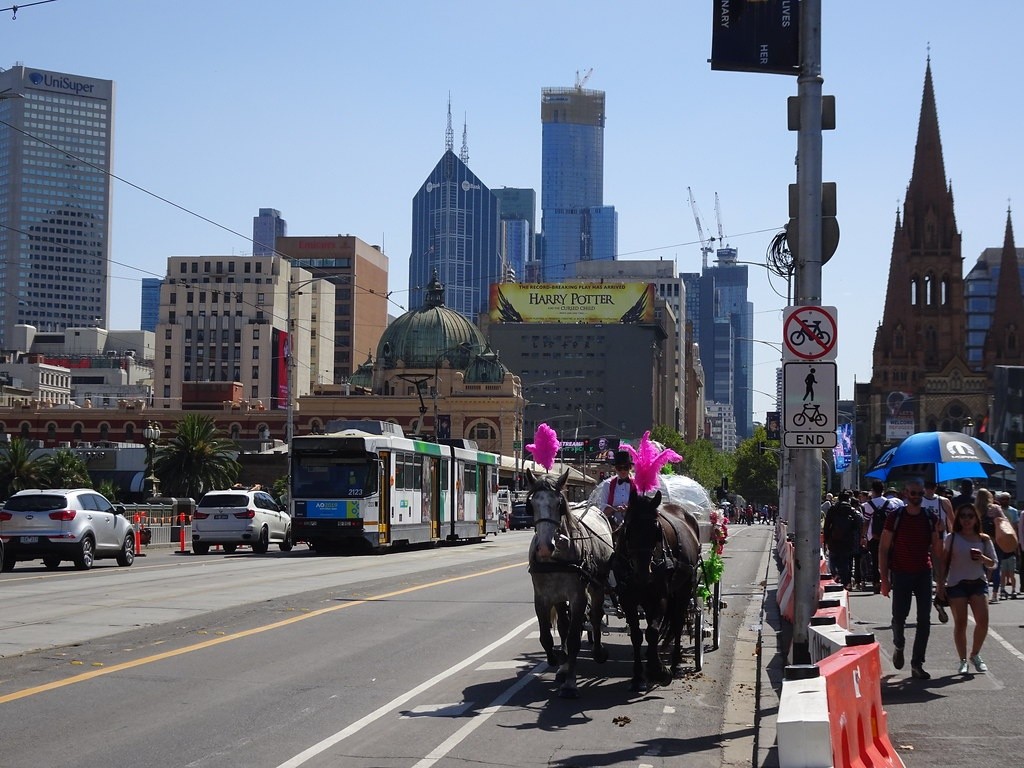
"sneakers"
969,654,989,672
958,657,969,674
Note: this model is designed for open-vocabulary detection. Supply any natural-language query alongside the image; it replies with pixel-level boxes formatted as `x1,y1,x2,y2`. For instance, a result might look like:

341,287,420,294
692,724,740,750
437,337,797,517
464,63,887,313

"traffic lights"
600,472,604,479
584,438,590,451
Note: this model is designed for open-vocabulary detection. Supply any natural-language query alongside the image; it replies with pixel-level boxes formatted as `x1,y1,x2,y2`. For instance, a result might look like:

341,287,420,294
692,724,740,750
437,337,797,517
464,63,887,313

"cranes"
575,67,594,87
715,192,729,249
687,186,714,268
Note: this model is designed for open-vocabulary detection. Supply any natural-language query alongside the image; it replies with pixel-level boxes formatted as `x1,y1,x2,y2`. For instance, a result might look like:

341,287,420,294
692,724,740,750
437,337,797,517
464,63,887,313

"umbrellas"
865,431,1017,519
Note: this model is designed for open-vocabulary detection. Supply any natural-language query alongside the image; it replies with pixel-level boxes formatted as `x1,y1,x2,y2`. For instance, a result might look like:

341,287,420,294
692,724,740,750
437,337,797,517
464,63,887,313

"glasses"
615,465,630,472
907,489,924,498
958,513,977,519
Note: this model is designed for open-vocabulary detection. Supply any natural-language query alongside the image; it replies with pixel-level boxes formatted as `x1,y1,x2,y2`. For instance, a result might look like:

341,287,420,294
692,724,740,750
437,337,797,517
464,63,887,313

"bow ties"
618,477,630,485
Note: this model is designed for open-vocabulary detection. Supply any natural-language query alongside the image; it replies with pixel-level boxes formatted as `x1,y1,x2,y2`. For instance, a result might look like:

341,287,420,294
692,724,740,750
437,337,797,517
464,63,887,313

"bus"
290,420,501,554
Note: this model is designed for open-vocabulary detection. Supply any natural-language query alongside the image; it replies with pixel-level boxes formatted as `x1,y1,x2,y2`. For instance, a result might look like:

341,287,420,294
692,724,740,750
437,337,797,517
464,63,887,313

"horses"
528,468,614,699
609,479,701,695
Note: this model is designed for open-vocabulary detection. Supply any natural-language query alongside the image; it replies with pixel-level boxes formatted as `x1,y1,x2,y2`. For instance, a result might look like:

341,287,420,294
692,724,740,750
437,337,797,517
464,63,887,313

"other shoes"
893,646,905,669
911,666,930,679
989,588,1024,604
833,575,883,592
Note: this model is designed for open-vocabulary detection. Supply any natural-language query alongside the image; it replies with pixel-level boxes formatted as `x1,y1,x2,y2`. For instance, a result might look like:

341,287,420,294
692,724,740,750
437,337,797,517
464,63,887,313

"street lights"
144,419,161,497
561,424,597,474
287,274,357,516
584,434,617,500
713,259,792,307
515,376,586,490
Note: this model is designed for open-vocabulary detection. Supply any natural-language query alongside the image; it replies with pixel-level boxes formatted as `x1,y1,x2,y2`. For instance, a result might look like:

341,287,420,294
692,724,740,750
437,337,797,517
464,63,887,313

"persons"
823,478,1024,602
596,438,614,459
725,505,777,526
938,503,999,674
600,452,640,525
878,478,943,680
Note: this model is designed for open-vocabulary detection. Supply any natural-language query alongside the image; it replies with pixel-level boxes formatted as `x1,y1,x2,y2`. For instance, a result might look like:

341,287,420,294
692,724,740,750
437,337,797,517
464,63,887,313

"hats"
611,451,635,466
883,487,899,496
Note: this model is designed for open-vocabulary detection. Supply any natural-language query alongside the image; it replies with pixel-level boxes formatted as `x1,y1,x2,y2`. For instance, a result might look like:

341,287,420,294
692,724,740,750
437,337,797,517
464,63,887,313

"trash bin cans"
171,526,181,542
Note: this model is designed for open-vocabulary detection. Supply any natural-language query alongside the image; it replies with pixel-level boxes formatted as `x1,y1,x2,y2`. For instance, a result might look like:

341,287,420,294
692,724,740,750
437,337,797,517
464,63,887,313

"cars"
509,504,535,531
499,506,508,533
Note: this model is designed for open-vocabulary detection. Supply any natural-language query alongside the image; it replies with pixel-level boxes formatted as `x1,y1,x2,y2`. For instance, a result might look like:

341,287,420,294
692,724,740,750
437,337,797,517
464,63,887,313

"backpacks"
828,504,859,552
868,500,892,536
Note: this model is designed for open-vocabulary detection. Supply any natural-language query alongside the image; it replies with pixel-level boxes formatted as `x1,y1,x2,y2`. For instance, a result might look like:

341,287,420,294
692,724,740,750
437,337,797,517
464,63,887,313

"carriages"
525,467,727,693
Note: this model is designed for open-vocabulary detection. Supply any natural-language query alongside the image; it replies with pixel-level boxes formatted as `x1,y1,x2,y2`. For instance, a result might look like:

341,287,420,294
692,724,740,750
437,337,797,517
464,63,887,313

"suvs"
191,489,294,555
0,488,135,572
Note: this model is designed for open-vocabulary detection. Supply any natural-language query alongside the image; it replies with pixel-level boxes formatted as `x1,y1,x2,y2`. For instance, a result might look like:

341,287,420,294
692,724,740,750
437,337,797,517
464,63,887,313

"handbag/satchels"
933,584,951,606
994,506,1019,553
